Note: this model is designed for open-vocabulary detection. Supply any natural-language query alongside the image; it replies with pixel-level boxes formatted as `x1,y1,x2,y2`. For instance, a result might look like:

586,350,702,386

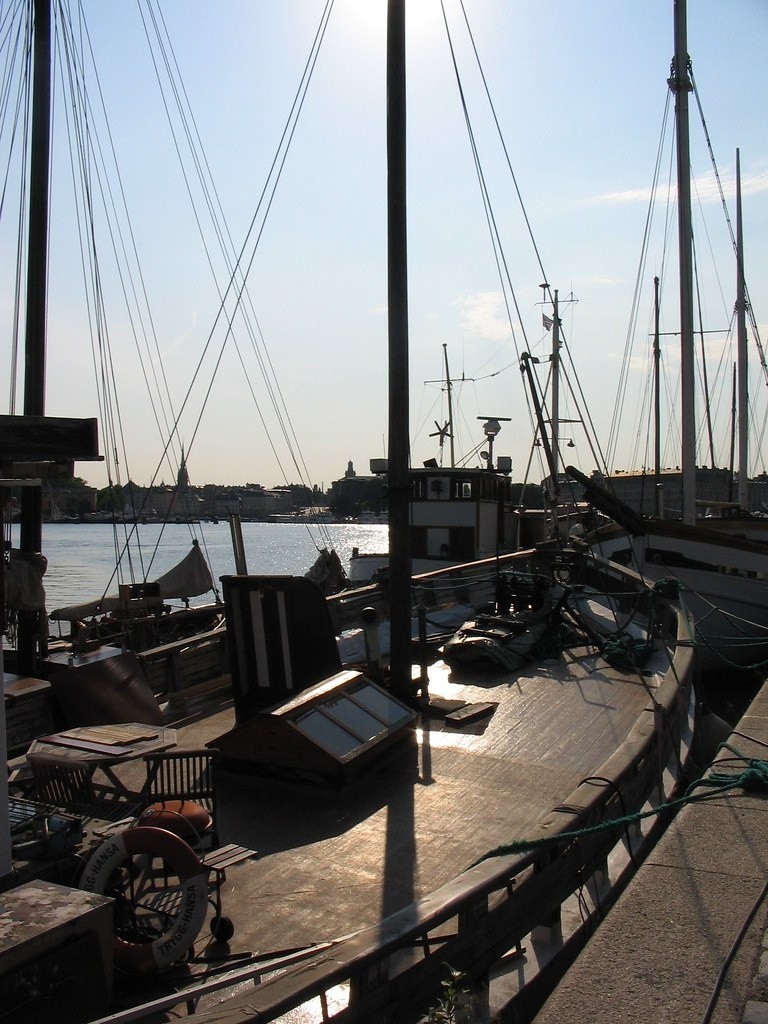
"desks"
0,879,116,1014
27,723,177,802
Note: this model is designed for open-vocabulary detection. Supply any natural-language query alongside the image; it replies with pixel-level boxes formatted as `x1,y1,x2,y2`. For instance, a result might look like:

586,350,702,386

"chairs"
24,754,143,825
137,748,227,882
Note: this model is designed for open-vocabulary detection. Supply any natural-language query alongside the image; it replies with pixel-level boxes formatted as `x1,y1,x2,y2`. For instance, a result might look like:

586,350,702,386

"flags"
543,314,553,331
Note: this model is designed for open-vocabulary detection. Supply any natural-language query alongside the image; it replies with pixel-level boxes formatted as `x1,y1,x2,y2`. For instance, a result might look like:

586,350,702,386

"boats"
348,281,610,584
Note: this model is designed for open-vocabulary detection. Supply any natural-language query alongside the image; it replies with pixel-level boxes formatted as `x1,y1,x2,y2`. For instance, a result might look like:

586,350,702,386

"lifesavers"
78,826,209,975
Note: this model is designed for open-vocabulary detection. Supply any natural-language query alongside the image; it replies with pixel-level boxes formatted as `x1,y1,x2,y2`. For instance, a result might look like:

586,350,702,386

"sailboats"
571,0,768,669
0,0,699,1024
0,0,343,767
